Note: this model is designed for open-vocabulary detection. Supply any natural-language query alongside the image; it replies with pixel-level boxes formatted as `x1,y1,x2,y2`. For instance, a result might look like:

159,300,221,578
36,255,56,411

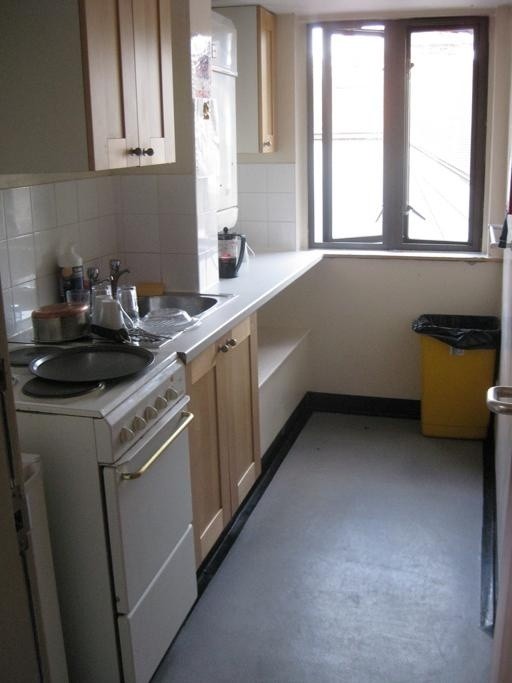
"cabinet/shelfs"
212,6,278,155
0,0,176,176
184,316,263,575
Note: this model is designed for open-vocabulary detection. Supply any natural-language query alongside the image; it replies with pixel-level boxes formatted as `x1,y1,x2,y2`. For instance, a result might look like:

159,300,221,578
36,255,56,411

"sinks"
137,291,240,320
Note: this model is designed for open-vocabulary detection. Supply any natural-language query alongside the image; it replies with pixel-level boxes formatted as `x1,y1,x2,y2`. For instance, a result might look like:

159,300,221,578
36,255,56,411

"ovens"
91,360,195,615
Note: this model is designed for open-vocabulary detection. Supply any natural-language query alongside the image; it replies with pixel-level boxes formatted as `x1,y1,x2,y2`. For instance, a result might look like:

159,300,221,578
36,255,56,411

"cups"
65,280,139,332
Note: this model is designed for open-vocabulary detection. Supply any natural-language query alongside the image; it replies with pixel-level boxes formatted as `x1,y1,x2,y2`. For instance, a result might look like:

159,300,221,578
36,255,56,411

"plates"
133,308,203,337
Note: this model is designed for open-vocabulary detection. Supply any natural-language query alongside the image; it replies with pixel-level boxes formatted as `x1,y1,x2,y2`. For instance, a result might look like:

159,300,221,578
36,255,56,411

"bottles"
56,236,84,300
217,226,246,279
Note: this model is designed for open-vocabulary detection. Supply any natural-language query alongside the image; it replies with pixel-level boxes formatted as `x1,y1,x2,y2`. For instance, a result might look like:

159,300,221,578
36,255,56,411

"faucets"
87,266,115,312
108,259,131,299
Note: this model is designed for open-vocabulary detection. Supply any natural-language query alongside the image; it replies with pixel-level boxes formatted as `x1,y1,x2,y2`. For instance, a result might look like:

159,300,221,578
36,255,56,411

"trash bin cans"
413,314,501,440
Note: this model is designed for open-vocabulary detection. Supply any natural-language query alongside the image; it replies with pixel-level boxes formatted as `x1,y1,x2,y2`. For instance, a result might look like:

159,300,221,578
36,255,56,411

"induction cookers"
6,342,181,416
